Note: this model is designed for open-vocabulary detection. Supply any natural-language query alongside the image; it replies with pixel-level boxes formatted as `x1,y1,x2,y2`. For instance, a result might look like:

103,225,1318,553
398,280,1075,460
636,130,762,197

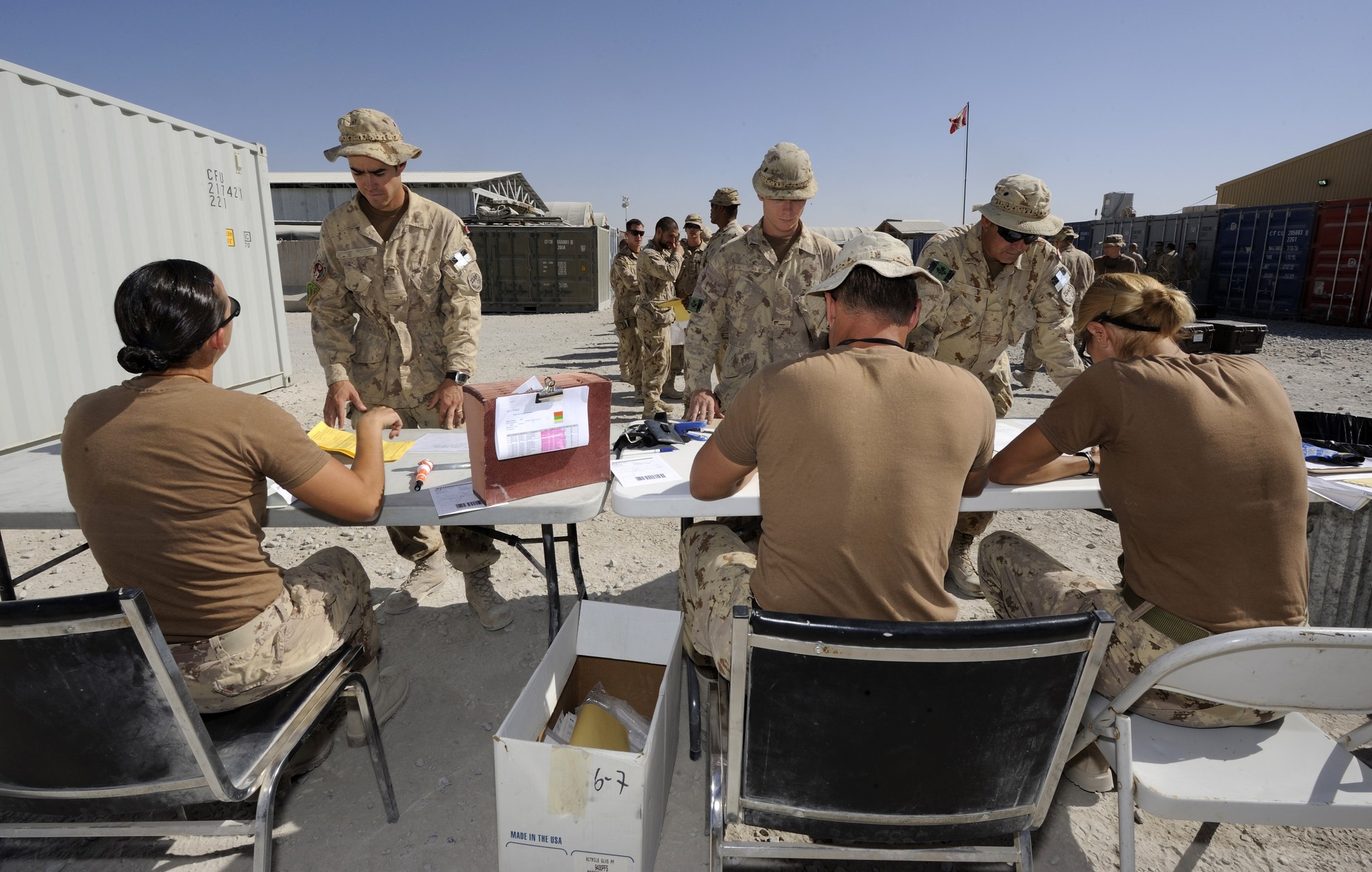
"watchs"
1075,452,1095,476
445,371,469,384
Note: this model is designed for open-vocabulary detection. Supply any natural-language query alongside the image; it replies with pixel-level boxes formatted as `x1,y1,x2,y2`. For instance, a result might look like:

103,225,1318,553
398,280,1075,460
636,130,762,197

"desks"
1,427,626,650
612,417,1372,761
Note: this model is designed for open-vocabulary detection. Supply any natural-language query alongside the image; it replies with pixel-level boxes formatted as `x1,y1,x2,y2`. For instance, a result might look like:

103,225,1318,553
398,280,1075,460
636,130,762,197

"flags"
948,104,966,134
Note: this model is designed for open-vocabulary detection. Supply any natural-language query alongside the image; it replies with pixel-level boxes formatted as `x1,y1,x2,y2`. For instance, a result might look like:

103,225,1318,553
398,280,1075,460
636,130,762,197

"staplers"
674,421,708,441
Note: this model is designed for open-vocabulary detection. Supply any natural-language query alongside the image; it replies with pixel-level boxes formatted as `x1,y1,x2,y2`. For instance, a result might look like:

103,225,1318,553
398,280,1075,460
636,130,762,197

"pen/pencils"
621,448,676,457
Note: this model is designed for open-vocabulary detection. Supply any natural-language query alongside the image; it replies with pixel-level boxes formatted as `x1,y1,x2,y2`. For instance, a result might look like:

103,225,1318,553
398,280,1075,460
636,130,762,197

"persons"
611,186,754,421
675,229,997,680
306,109,514,631
59,258,411,781
912,175,1087,600
1013,226,1208,391
978,273,1308,794
683,141,843,425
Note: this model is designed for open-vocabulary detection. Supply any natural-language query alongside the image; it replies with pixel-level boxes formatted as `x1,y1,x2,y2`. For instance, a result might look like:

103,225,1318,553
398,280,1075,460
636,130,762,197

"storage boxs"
1045,196,1372,329
912,235,935,266
457,213,628,314
0,57,296,457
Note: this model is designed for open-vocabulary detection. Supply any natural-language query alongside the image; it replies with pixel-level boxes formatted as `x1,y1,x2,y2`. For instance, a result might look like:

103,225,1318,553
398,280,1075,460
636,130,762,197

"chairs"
0,584,400,871
1065,626,1371,872
705,604,1116,872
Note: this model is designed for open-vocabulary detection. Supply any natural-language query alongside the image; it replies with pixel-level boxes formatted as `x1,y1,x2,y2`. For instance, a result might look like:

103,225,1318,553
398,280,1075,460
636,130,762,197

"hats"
1099,234,1126,247
752,142,819,200
323,109,422,166
803,231,944,333
709,187,741,206
973,174,1064,236
700,226,712,239
1048,226,1079,241
683,214,704,230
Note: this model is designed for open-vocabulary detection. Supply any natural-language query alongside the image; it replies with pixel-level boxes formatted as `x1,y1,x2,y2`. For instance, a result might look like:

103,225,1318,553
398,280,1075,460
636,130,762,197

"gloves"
1302,441,1365,466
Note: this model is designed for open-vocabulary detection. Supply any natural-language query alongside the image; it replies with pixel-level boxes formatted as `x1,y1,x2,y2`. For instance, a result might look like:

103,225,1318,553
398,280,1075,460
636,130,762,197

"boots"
383,549,446,615
244,724,333,802
1012,369,1037,388
694,665,718,752
1062,723,1115,791
463,566,514,631
345,655,410,749
620,365,690,420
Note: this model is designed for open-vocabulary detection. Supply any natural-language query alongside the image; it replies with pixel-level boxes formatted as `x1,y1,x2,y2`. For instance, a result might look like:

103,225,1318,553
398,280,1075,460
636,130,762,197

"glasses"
710,203,715,208
627,229,645,237
197,295,240,351
1078,333,1093,365
997,225,1040,245
685,224,700,228
1137,245,1188,249
